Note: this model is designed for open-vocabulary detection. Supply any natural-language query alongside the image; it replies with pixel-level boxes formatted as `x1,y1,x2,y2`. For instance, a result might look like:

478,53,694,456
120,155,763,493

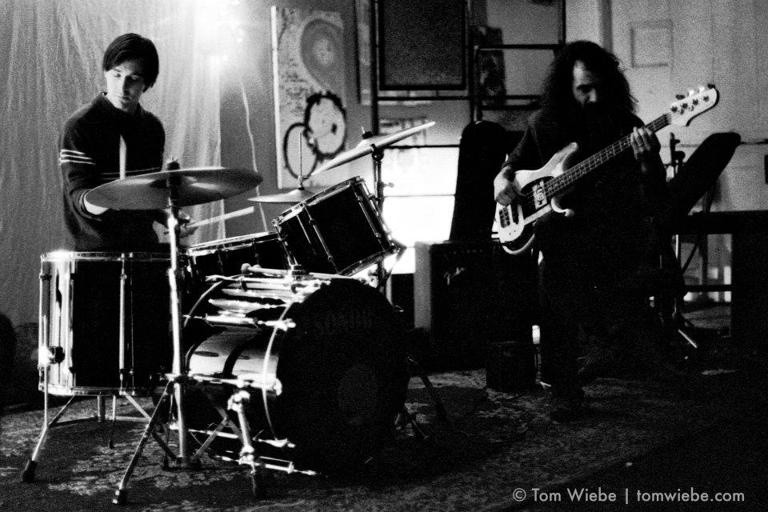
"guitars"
490,84,719,256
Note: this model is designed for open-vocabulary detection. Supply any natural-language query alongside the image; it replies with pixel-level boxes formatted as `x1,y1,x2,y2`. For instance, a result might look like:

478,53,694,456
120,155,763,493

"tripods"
21,395,181,483
372,151,458,452
112,197,267,504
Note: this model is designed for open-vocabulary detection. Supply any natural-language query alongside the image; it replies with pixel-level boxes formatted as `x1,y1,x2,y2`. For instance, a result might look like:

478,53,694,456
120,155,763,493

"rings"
638,143,644,147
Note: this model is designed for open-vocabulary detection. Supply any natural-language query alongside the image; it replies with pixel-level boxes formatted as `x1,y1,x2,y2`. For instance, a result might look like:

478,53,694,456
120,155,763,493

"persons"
482,39,670,399
57,31,196,250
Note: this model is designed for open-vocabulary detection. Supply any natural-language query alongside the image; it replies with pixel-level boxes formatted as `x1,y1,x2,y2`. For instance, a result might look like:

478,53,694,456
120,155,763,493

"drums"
189,176,403,476
37,248,190,396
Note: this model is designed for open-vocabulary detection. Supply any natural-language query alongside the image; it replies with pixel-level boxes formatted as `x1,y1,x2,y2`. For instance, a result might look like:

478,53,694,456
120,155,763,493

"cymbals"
86,168,263,210
310,122,435,174
